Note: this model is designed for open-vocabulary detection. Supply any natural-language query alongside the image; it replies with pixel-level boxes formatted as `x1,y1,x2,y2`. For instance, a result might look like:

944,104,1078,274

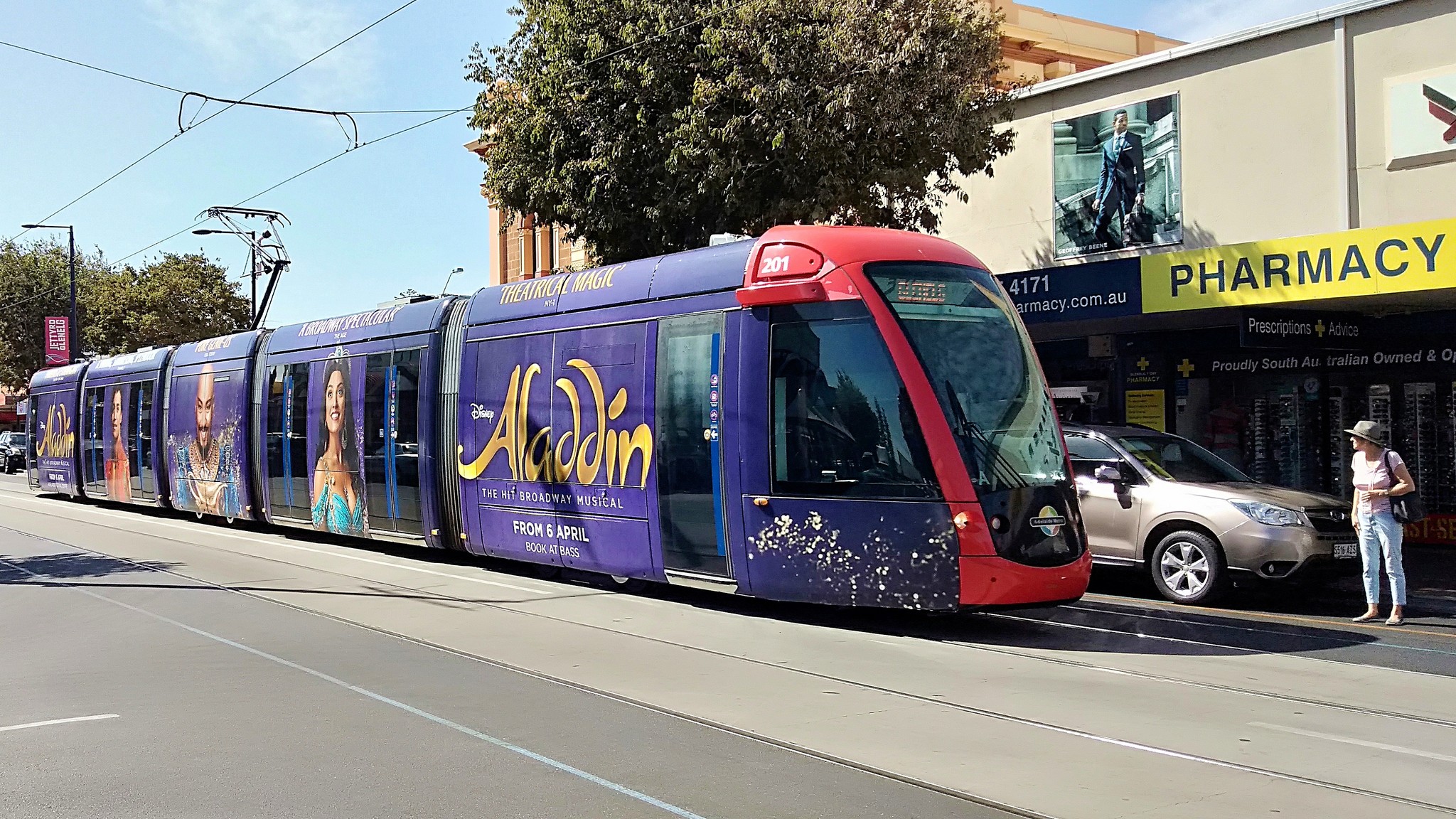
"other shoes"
1352,612,1381,622
1386,616,1405,625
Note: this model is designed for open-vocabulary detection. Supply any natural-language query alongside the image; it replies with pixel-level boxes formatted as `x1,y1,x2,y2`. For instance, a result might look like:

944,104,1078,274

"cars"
982,428,1362,605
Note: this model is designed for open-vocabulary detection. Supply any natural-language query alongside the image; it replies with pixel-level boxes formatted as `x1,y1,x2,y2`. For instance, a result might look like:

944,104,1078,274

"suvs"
0,431,38,475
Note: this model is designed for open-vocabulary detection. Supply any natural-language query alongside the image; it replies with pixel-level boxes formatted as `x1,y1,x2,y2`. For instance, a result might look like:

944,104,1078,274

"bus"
22,205,1091,616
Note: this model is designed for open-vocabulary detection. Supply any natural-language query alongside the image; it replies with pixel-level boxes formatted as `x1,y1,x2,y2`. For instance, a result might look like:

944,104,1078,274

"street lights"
21,223,77,364
192,229,257,328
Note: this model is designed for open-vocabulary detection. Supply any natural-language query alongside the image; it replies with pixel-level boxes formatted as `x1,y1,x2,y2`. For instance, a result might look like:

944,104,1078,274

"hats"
1343,421,1383,445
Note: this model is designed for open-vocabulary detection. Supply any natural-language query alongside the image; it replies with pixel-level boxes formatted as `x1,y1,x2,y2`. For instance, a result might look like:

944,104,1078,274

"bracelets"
1384,489,1389,495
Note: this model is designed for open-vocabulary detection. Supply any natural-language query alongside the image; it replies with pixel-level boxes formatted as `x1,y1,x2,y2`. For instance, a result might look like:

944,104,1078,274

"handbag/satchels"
1384,450,1411,524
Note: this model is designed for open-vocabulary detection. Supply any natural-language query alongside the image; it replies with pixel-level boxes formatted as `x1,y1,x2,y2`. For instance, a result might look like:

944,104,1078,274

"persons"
177,363,240,517
312,360,365,537
1344,421,1415,626
105,379,133,503
1092,109,1145,252
